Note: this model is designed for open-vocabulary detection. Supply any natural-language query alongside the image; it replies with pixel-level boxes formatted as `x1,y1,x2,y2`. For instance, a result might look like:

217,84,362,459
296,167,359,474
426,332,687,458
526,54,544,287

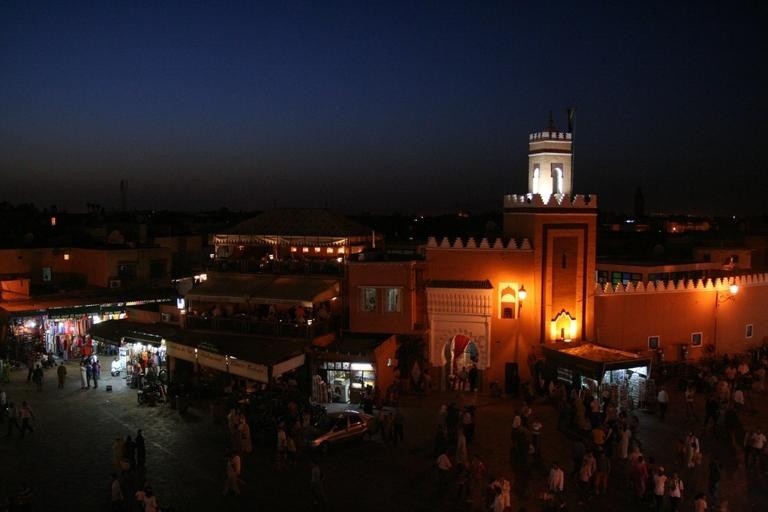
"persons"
5,400,37,438
2,306,98,391
109,430,157,510
219,306,768,510
128,356,166,405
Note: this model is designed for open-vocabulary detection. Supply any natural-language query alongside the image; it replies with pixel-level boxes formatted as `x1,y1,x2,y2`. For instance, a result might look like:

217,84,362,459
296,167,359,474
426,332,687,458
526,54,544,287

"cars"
292,410,373,450
